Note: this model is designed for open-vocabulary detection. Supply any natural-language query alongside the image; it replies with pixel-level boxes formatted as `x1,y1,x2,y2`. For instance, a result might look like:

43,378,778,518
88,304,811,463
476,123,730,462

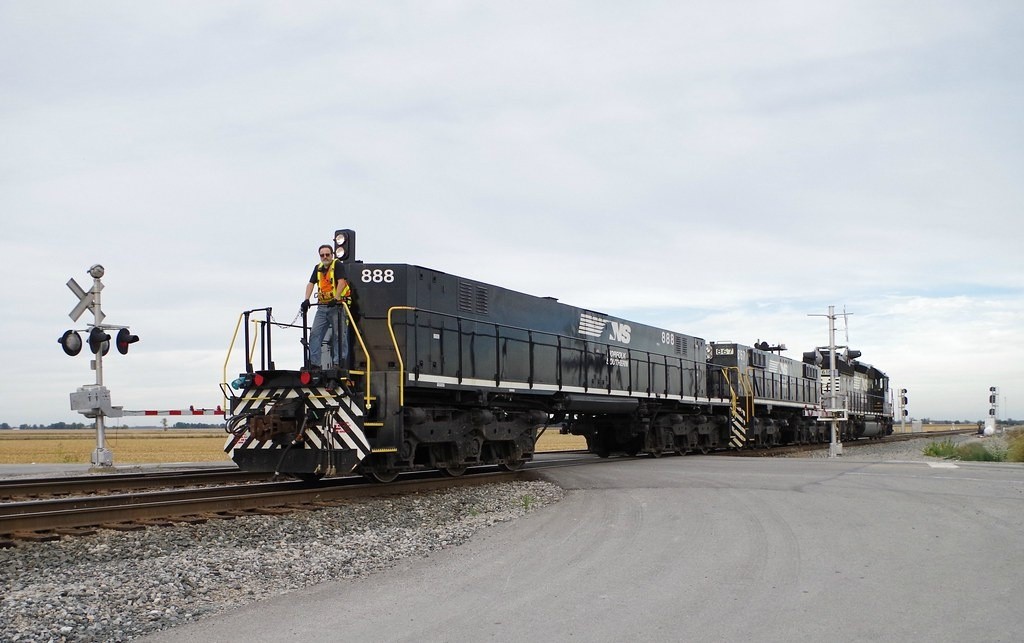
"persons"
301,244,351,371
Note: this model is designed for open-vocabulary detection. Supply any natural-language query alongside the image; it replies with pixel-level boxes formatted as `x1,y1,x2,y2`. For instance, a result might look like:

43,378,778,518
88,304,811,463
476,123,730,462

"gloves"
327,298,338,308
301,299,311,314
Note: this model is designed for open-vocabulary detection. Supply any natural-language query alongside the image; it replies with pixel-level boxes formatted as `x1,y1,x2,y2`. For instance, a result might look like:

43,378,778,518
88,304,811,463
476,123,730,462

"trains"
220,229,894,485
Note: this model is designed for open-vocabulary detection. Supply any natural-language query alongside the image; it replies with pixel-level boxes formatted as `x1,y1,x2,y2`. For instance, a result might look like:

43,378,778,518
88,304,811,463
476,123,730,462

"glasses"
319,253,331,258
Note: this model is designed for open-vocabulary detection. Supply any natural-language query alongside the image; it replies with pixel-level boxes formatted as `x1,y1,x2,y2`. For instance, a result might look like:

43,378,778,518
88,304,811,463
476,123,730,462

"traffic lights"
117,328,139,355
90,327,111,353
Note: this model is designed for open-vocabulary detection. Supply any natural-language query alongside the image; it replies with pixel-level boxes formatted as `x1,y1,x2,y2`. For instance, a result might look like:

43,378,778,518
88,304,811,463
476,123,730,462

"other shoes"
330,364,339,369
301,364,321,371
